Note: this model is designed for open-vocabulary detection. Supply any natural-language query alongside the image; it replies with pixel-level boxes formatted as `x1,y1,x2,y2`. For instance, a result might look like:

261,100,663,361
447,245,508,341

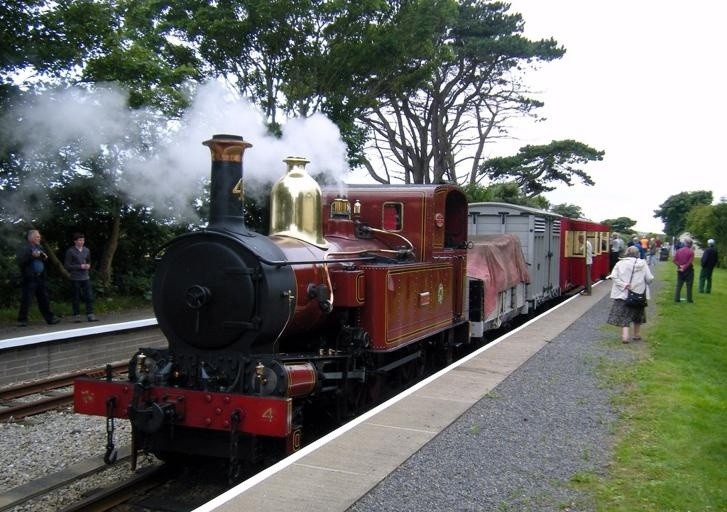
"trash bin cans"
660,248,667,260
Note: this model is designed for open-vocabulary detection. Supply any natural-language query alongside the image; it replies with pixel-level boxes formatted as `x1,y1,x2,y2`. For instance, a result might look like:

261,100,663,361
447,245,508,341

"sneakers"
17,321,27,326
73,314,97,322
580,290,591,296
47,318,60,324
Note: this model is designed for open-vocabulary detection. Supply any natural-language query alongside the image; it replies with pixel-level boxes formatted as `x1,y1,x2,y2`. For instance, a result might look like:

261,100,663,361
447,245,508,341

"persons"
607,245,654,344
578,235,593,296
16,229,61,327
697,239,718,293
672,239,695,303
609,232,662,273
64,232,98,323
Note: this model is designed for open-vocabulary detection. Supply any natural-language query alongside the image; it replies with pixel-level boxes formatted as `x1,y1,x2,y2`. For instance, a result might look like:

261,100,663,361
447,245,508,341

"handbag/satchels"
625,290,647,308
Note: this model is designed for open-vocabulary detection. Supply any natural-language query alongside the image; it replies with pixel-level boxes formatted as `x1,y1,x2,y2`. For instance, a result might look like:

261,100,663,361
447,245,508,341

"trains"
73,134,610,482
